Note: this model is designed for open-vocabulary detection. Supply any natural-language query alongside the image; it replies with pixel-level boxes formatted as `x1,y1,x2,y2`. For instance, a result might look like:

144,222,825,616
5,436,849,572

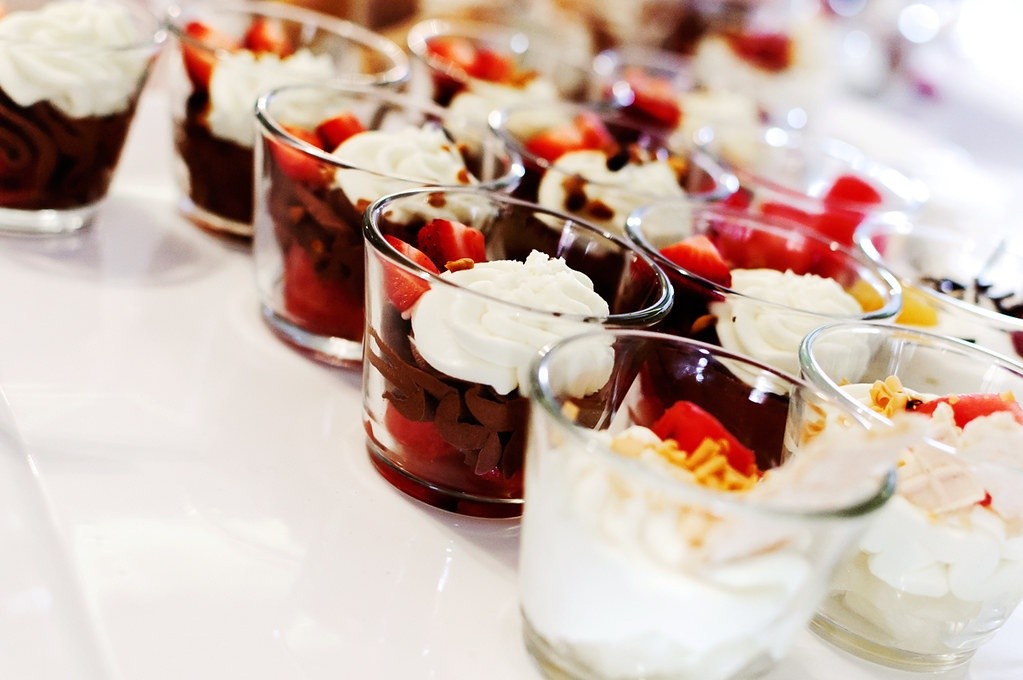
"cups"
3,0,1022,674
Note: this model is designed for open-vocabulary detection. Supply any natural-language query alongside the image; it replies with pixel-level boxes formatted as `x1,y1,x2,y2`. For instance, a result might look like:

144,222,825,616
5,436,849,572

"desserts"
0,0,1020,676
